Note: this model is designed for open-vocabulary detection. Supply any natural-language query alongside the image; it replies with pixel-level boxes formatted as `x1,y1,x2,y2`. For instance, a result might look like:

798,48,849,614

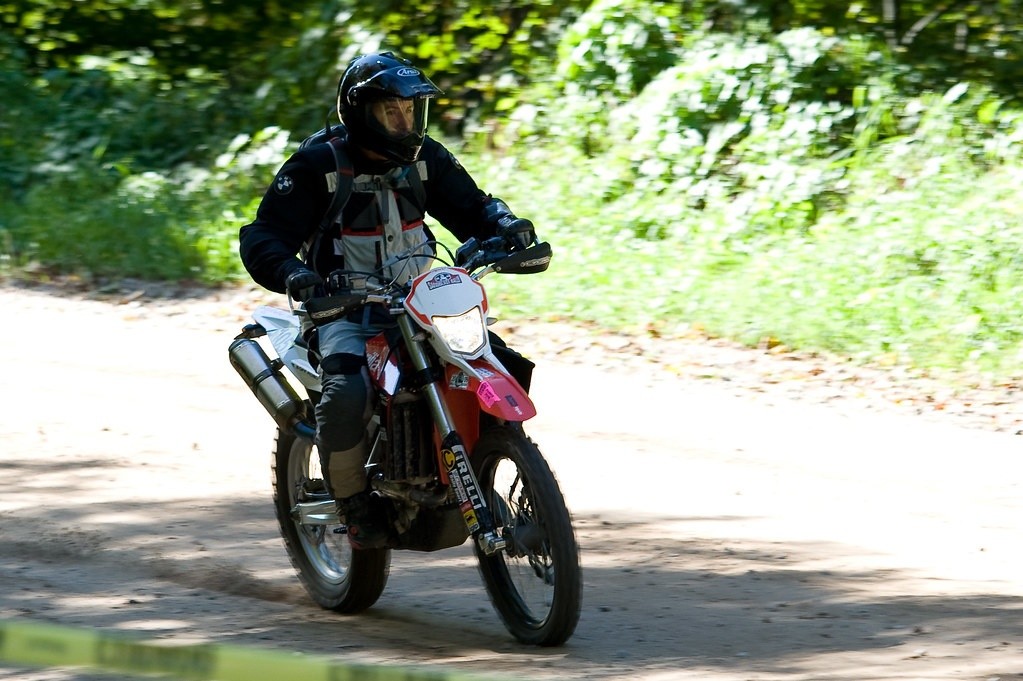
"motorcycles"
227,230,584,650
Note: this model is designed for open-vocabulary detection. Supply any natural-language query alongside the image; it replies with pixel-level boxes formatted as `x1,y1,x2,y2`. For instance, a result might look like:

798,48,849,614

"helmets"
336,51,445,162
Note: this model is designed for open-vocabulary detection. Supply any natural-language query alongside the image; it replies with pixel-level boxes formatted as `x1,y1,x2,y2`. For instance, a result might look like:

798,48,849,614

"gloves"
479,203,536,254
284,267,323,302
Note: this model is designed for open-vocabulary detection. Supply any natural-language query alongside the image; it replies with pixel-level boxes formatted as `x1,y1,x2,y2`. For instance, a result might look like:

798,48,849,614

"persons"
238,53,537,551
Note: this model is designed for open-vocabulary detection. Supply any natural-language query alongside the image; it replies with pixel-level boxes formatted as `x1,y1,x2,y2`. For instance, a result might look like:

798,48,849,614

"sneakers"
493,491,513,526
334,490,389,549
